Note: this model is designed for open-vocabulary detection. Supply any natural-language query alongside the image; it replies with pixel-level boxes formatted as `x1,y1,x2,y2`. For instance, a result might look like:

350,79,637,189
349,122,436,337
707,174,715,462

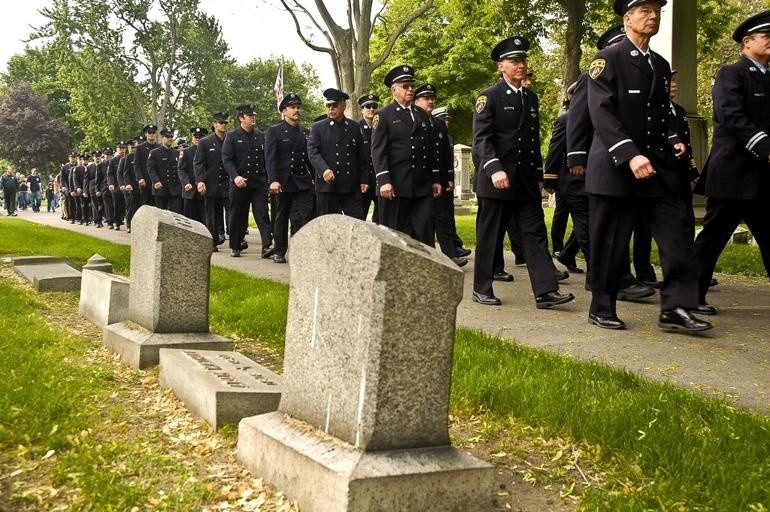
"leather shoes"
212,229,286,263
449,247,717,331
61,216,130,233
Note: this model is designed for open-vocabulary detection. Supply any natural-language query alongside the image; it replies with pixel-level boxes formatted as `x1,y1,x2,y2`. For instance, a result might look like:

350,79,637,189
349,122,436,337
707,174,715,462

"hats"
733,10,770,43
68,125,156,158
323,89,349,104
595,24,626,51
191,105,258,136
279,93,301,112
160,128,175,137
384,65,416,87
358,94,379,109
413,84,436,100
177,140,189,147
491,36,529,62
431,107,448,117
614,0,667,16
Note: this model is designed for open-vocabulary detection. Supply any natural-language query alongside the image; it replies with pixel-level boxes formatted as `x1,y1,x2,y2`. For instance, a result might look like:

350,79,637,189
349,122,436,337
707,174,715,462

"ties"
517,90,522,95
405,108,412,120
645,54,650,63
670,107,675,117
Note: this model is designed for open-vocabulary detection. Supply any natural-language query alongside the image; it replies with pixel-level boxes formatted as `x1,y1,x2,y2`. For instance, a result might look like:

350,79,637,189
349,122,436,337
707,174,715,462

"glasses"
326,103,337,107
366,106,377,109
403,84,413,89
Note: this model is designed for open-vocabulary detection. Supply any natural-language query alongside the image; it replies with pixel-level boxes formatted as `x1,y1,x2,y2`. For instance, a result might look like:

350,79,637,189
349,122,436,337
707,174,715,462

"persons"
469,37,574,308
545,2,770,333
0,166,60,216
54,112,230,252
221,64,471,267
